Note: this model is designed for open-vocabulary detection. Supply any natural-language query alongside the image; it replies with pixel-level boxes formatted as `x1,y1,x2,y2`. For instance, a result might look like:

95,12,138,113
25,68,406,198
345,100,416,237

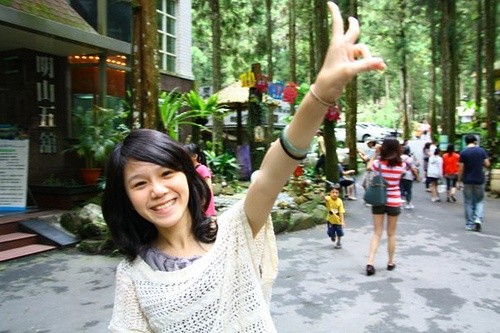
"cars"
302,123,399,169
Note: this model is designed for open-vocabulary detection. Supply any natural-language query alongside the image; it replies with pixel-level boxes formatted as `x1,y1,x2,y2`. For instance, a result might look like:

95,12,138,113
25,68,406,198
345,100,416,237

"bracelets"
309,82,338,109
278,125,312,161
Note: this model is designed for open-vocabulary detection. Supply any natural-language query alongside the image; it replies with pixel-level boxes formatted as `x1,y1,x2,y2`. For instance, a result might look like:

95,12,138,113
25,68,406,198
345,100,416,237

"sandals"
386,263,397,271
365,264,377,276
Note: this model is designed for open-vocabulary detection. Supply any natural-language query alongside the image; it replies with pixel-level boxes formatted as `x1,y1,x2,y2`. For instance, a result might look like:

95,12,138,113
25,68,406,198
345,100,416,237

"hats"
365,136,376,144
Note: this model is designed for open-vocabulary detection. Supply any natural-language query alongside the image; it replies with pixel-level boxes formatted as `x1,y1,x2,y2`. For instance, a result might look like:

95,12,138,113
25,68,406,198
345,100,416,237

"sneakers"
336,243,342,249
331,237,336,241
344,194,358,200
446,193,457,202
431,197,442,203
404,204,415,210
464,219,482,232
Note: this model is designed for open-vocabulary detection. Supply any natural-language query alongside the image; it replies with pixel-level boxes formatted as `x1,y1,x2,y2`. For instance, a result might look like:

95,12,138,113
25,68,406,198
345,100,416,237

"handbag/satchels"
363,163,387,206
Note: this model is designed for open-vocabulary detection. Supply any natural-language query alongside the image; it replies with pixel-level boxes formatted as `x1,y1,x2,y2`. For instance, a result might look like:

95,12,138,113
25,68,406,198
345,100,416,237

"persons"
182,143,215,218
320,183,347,249
314,117,460,210
363,137,405,276
100,0,386,333
457,132,490,232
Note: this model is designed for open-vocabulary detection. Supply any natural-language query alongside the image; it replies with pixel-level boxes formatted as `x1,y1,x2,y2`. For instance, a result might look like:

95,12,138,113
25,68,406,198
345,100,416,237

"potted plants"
61,105,125,185
203,150,225,196
490,163,500,195
